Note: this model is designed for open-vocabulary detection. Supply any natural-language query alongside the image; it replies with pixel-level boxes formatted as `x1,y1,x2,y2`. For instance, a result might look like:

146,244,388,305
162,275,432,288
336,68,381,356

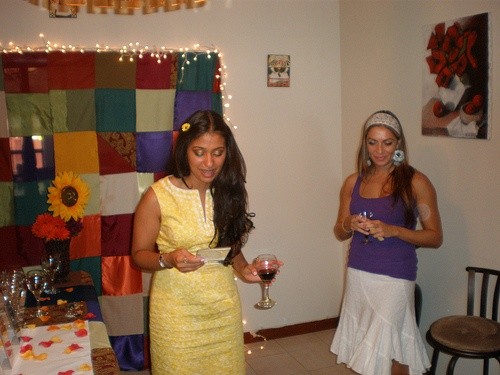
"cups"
0,268,24,322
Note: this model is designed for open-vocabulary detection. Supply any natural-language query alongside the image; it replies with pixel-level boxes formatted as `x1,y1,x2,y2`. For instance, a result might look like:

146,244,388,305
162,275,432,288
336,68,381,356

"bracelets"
341,221,351,232
159,255,165,268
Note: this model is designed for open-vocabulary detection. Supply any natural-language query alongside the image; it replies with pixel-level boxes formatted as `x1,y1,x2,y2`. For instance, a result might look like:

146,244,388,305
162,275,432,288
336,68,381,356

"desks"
0,272,121,375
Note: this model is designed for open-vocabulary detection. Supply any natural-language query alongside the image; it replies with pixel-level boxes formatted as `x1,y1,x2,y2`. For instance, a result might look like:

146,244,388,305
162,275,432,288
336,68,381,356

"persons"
330,111,443,375
132,111,282,375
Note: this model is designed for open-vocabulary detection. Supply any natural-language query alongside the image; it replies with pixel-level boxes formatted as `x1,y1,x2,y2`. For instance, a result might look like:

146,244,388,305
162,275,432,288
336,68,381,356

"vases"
45,238,70,281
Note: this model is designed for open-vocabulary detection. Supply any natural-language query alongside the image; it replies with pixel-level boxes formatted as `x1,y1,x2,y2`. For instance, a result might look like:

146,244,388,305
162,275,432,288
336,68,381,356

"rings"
185,258,186,263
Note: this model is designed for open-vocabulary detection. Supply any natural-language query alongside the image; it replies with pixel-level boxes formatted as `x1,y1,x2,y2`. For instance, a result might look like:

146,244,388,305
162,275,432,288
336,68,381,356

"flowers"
31,171,88,240
182,123,190,131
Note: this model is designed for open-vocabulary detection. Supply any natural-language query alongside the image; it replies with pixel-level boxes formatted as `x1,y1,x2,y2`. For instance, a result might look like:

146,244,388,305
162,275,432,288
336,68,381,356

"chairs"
425,266,500,375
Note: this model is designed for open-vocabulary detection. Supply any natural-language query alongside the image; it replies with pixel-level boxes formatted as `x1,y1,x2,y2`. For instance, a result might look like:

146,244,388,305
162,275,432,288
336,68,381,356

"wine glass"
26,270,48,318
41,252,63,294
255,254,278,309
359,210,376,244
